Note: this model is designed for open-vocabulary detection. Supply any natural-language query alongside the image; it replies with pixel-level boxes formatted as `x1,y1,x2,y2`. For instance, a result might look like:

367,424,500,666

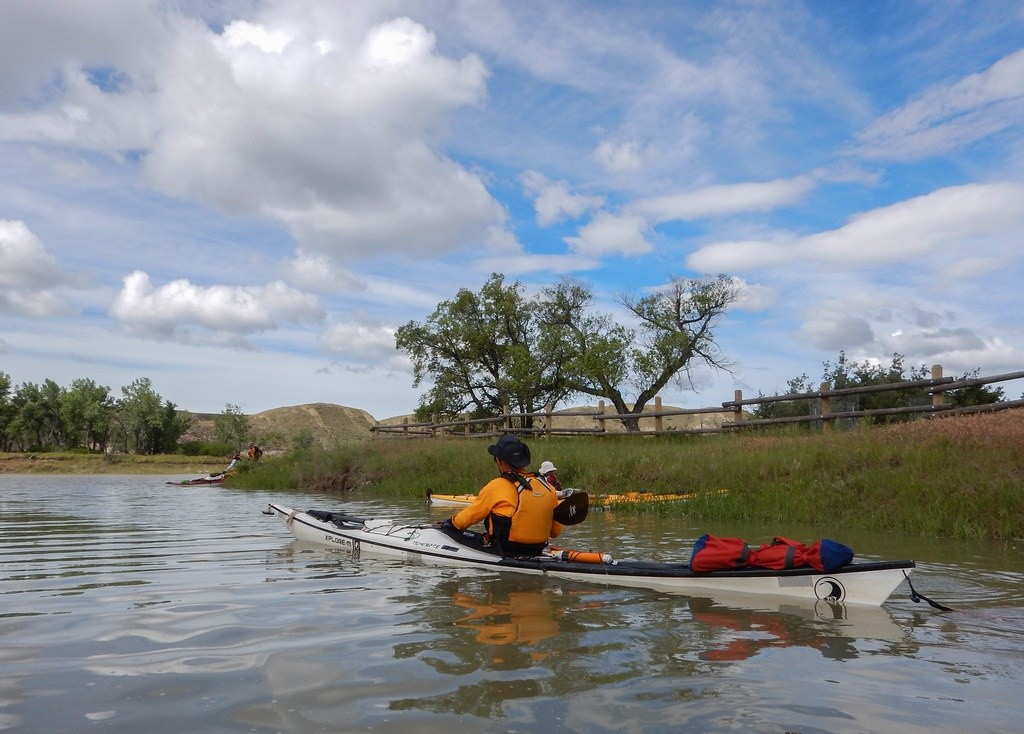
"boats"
425,486,731,512
165,474,225,486
263,503,916,606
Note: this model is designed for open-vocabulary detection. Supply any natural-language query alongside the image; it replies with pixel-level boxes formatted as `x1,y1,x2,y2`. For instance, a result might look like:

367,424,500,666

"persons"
437,435,563,556
539,460,564,491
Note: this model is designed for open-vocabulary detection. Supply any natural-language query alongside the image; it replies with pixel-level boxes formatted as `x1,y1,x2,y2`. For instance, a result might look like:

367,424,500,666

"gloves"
442,518,452,529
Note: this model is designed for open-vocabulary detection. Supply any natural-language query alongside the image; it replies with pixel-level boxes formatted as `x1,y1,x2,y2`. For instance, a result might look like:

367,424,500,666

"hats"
539,461,557,475
248,442,254,447
488,435,531,468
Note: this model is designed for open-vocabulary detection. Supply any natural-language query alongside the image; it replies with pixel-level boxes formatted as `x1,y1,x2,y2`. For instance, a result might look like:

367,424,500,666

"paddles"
553,491,589,525
